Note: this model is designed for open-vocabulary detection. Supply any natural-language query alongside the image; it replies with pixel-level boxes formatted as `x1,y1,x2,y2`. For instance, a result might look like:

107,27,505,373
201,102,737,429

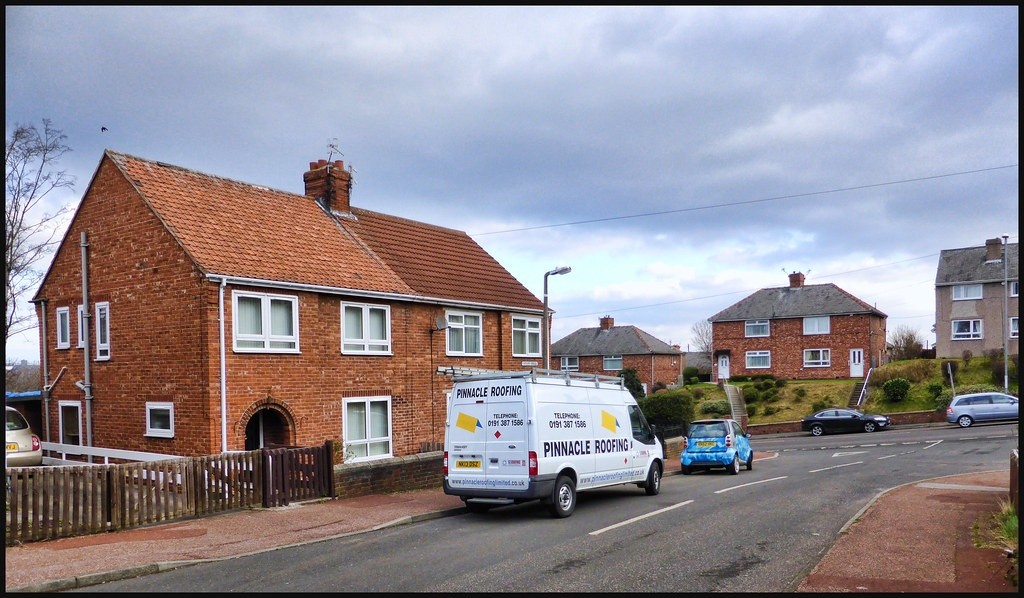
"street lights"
1002,233,1009,393
543,265,572,374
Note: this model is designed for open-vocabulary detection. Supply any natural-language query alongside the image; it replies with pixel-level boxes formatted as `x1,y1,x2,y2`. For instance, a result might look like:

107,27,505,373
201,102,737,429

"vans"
442,367,666,517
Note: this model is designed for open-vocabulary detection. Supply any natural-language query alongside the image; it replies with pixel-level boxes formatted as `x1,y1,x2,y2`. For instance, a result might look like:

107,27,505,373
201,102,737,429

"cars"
947,392,1018,428
800,408,891,436
680,419,754,475
6,406,43,467
